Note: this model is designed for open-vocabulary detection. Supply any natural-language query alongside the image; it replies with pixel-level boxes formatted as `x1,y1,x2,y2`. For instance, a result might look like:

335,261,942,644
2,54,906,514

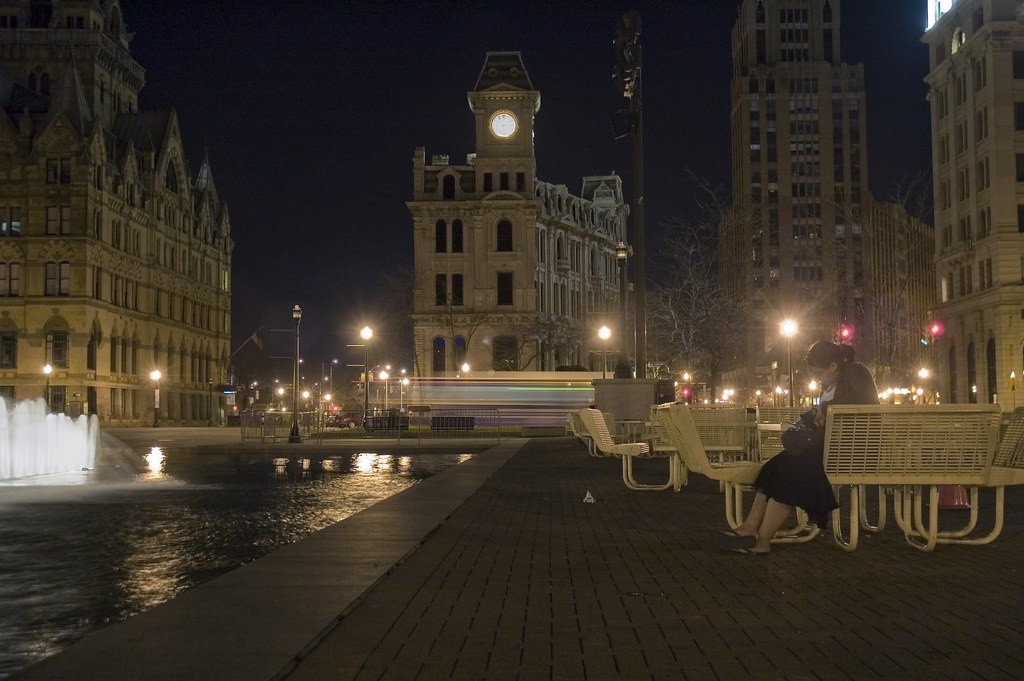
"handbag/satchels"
781,408,826,456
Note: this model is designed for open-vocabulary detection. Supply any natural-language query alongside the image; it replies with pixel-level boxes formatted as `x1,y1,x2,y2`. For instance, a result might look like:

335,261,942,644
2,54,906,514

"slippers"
719,528,771,555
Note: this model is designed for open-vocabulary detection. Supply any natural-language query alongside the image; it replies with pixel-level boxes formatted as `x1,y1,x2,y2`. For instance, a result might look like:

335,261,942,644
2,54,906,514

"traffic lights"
840,325,852,341
930,324,939,335
683,387,690,397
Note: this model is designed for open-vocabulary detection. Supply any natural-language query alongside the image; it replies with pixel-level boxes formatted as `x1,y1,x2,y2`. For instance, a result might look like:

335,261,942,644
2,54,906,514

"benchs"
430,417,475,432
570,403,1024,551
364,417,409,432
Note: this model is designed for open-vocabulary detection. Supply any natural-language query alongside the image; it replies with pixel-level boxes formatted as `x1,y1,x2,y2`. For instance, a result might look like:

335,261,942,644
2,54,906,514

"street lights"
289,304,303,442
399,368,406,411
330,359,338,401
598,326,611,378
43,363,52,411
277,388,284,413
303,390,310,433
781,319,798,407
150,369,163,428
614,241,635,378
919,368,929,403
385,364,391,412
400,378,409,411
361,326,374,427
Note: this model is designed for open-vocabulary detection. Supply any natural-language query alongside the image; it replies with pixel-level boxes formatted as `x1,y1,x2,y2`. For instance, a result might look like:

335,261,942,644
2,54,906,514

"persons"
708,340,881,556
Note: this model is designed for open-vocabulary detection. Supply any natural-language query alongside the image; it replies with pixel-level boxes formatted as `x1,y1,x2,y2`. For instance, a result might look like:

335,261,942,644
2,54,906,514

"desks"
644,422,664,427
757,423,794,431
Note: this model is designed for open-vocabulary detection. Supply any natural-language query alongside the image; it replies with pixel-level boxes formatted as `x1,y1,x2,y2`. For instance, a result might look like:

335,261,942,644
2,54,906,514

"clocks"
491,114,515,137
